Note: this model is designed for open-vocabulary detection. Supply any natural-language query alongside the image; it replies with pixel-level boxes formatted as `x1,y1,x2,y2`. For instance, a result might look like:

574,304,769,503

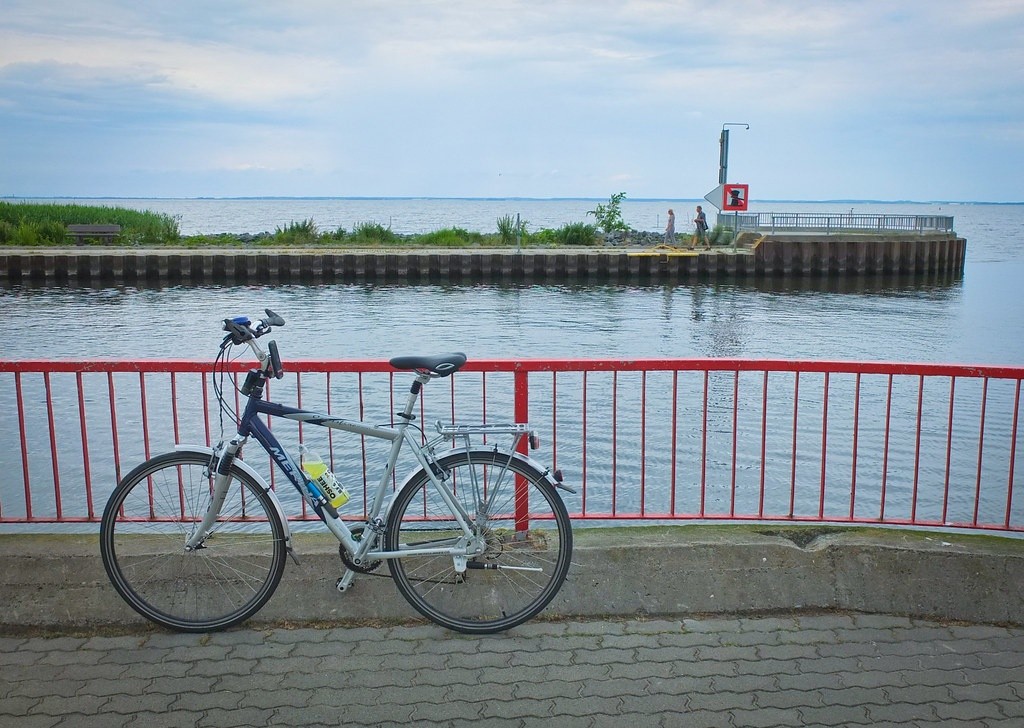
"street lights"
719,122,749,212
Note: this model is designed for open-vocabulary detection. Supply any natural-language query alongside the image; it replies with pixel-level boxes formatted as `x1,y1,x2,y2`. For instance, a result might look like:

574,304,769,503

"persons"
687,206,711,251
660,209,677,249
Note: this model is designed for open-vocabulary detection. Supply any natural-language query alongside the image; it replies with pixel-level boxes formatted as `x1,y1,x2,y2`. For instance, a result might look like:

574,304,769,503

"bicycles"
99,308,577,635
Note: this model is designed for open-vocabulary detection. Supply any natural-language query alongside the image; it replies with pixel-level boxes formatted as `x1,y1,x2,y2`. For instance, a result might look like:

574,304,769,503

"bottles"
296,443,351,509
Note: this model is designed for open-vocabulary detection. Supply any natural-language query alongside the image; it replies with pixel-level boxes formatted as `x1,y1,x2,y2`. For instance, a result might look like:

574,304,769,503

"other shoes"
704,247,711,251
687,247,694,251
